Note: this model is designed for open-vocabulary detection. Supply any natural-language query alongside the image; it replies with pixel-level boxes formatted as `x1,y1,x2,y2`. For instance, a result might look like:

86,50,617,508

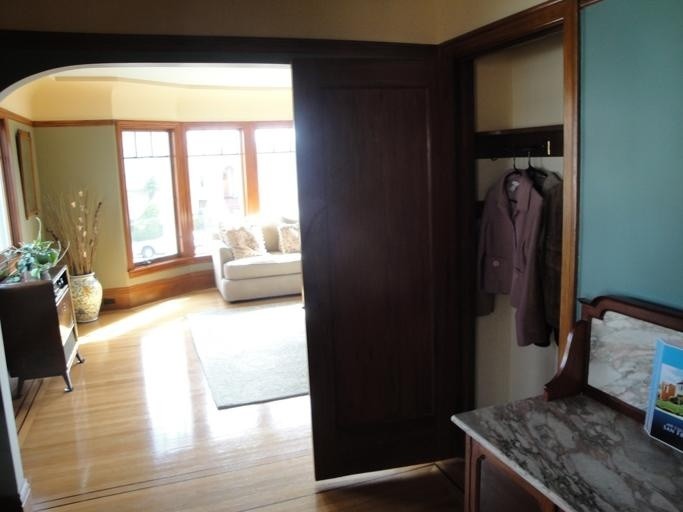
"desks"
450,394,683,511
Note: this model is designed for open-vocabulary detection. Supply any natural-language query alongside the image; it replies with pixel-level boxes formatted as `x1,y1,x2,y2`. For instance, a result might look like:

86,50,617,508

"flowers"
38,189,104,276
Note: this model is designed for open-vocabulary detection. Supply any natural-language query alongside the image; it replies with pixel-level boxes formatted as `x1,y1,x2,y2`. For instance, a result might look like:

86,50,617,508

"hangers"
489,156,544,188
516,150,561,184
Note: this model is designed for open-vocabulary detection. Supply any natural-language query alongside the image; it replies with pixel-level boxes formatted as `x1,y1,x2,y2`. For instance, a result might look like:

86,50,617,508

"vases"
66,272,103,323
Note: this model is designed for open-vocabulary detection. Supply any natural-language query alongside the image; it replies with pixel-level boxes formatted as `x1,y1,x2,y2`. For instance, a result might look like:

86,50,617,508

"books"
643,336,682,453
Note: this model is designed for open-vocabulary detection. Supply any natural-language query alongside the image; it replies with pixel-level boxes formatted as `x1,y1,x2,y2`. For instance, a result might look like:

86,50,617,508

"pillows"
277,223,300,253
220,225,268,257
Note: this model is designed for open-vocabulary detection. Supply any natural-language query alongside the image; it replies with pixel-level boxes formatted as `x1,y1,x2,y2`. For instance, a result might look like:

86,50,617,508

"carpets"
184,302,309,409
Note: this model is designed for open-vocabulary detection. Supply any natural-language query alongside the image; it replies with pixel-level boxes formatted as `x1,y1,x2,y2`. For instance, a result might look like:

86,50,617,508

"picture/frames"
16,129,39,219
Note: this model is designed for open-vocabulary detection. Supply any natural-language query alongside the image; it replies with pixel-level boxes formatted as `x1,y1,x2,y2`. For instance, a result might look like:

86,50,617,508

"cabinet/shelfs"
0,264,85,401
291,1,579,481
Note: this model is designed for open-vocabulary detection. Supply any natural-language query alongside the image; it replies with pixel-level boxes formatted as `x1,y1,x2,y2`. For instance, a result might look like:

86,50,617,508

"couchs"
209,219,304,302
